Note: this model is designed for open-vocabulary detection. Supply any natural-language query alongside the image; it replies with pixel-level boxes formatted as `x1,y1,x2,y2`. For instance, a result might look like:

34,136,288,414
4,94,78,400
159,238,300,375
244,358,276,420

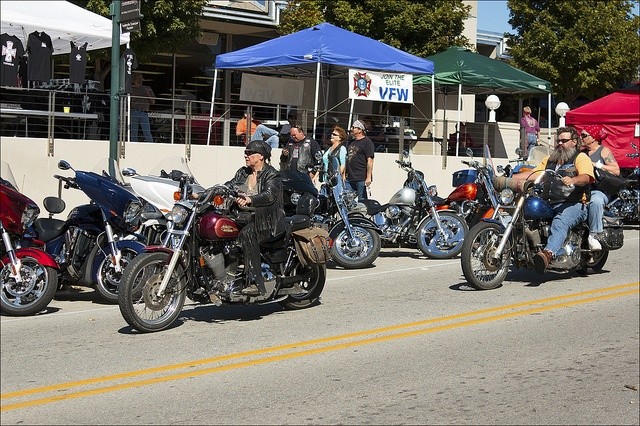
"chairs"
280,192,317,232
33,195,69,244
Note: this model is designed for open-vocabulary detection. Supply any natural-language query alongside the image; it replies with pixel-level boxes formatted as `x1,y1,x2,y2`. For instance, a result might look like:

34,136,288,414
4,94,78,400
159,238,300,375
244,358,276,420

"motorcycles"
0,159,61,316
117,169,327,333
277,149,384,270
115,167,169,246
356,148,469,258
14,159,154,303
427,144,496,232
607,142,639,229
457,146,611,290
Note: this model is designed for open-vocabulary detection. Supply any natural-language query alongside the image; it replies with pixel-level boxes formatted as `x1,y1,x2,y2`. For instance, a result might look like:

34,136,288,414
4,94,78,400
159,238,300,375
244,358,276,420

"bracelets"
595,159,603,169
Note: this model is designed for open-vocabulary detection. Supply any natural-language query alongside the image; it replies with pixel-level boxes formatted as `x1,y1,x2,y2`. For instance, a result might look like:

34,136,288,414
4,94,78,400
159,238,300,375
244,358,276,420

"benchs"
1,106,99,139
123,108,290,146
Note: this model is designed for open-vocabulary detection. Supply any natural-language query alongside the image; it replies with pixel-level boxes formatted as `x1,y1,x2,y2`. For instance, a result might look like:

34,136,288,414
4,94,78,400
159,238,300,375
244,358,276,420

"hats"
523,106,531,113
132,73,143,85
584,124,608,141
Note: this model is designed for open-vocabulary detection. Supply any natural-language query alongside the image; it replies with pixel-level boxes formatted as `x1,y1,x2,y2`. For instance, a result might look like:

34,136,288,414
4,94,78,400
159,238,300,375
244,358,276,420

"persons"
345,119,374,200
235,108,281,148
131,73,156,142
280,124,323,179
312,126,347,185
511,127,595,274
189,140,287,296
449,122,490,156
520,106,540,156
579,125,620,252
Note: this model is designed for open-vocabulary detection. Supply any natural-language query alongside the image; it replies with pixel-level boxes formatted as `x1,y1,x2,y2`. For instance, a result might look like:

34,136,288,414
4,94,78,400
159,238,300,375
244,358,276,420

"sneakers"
588,235,602,251
242,284,262,295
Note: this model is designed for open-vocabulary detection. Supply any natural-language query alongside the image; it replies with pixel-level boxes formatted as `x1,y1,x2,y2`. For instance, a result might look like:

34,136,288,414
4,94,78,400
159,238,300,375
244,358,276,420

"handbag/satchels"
594,169,624,193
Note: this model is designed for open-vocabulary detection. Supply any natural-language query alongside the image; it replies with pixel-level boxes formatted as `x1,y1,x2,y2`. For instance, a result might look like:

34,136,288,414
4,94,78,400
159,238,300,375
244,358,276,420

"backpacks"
291,227,332,266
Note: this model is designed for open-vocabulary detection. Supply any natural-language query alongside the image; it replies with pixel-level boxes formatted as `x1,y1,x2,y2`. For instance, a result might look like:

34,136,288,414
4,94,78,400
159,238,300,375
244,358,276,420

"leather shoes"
534,250,551,274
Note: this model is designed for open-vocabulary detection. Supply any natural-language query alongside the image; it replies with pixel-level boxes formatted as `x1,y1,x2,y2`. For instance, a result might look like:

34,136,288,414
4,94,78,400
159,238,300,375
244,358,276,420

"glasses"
581,133,589,138
244,150,258,155
351,126,359,129
556,139,571,144
331,133,339,136
